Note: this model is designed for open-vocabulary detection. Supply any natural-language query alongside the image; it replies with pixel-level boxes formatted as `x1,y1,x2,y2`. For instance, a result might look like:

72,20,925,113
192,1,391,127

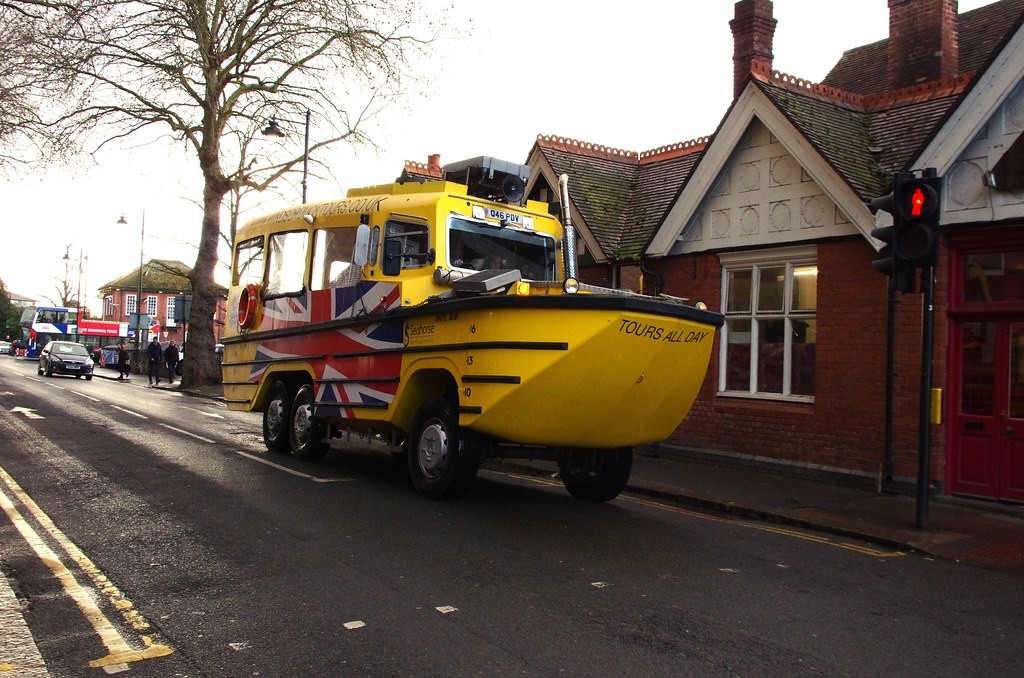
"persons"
164,339,180,383
148,337,162,385
117,343,130,379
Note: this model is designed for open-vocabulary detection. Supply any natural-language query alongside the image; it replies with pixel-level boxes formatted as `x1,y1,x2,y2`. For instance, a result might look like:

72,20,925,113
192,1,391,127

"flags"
251,279,403,420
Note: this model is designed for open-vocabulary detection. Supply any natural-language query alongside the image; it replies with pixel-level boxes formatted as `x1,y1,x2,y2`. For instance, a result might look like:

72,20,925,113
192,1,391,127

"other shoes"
169,378,175,384
150,379,161,384
119,373,129,378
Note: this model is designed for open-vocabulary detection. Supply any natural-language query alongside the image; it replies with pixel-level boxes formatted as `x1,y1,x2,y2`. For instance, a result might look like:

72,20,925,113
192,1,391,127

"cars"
0,340,34,356
37,340,95,380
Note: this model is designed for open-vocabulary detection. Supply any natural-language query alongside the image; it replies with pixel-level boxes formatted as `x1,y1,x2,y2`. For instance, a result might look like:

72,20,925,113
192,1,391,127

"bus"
15,306,107,363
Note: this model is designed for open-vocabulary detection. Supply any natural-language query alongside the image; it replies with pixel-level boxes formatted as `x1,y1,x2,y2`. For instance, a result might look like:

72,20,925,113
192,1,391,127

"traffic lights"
869,172,915,295
901,177,942,268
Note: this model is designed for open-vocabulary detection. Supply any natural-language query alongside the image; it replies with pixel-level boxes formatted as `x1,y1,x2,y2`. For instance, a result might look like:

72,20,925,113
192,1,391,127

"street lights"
62,242,83,308
117,209,146,373
261,108,311,206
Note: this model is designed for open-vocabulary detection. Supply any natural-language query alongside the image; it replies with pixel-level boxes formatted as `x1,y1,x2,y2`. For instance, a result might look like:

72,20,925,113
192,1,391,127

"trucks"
217,151,727,510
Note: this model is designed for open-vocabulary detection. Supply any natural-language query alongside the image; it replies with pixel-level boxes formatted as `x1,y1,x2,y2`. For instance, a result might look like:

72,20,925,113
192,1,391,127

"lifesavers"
237,284,257,329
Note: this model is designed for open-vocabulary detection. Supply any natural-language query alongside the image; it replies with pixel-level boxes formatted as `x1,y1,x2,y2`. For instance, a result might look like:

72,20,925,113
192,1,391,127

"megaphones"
495,174,526,203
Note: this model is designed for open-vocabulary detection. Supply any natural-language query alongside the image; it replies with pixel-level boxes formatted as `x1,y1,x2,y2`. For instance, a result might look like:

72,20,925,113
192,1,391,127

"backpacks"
125,351,130,360
